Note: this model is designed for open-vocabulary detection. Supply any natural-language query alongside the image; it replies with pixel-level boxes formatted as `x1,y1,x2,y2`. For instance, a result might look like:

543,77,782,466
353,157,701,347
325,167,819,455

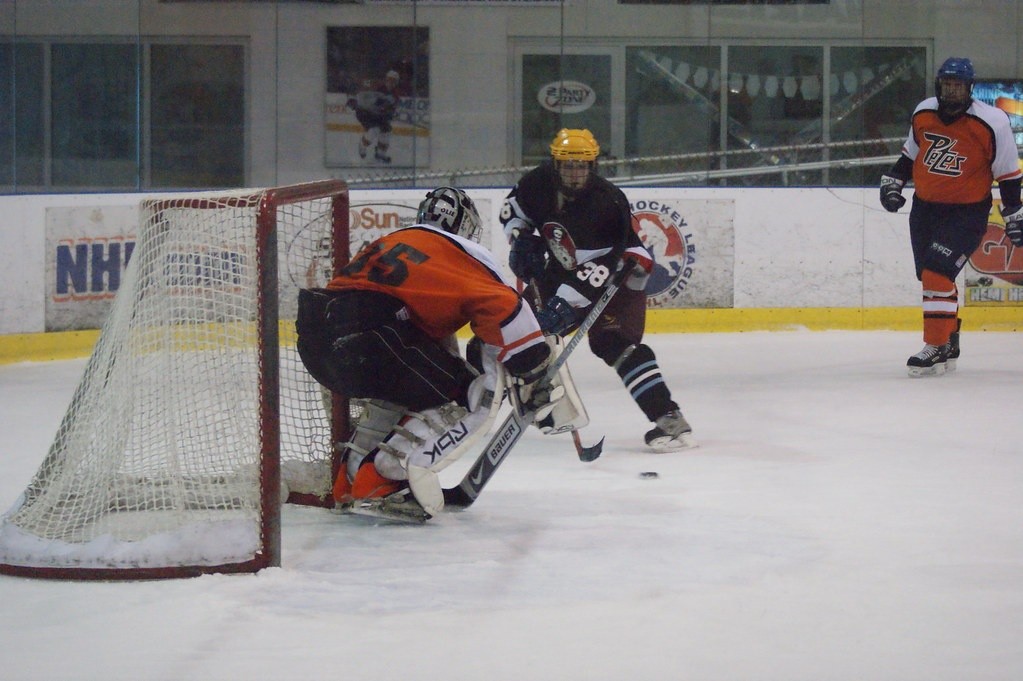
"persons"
357,70,400,164
500,127,699,453
786,55,823,119
293,186,564,525
880,57,1023,378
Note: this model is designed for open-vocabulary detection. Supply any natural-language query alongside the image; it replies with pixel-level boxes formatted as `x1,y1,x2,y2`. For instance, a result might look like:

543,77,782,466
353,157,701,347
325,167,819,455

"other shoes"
347,463,432,525
329,462,357,514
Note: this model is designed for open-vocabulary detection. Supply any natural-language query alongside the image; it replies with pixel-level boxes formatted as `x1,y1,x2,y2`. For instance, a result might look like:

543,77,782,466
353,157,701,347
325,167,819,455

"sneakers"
357,142,367,160
374,146,391,165
945,318,962,371
907,343,947,377
645,409,699,453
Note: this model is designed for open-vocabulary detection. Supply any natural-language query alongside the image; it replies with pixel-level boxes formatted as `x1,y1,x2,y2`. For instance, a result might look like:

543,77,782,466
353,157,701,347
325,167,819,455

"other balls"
641,472,658,479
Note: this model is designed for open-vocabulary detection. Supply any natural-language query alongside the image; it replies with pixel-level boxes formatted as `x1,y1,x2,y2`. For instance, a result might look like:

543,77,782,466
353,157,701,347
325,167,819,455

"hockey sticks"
396,256,636,510
529,279,605,463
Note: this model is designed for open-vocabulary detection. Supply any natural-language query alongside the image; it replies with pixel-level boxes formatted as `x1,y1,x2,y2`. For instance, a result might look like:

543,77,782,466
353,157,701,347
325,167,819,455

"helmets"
416,185,483,244
382,70,399,90
935,57,977,115
549,127,600,193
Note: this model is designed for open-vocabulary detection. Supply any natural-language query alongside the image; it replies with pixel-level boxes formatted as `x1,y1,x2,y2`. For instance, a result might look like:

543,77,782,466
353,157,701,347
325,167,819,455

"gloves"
508,230,546,285
536,295,579,336
511,363,555,435
880,174,907,212
1001,204,1023,248
391,105,401,117
345,98,357,111
